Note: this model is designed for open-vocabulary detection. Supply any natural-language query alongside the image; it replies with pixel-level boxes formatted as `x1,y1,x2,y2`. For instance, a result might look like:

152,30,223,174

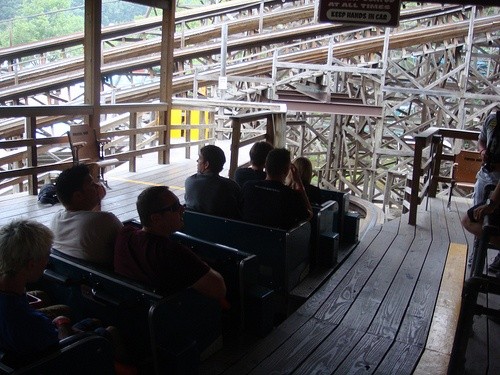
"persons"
184,145,240,218
467,110,500,267
52,163,124,264
234,141,273,187
241,148,313,231
0,219,123,375
461,179,500,274
113,186,226,298
287,158,325,206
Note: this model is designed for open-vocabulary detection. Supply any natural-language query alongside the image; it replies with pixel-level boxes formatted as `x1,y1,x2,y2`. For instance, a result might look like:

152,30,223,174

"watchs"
484,196,495,207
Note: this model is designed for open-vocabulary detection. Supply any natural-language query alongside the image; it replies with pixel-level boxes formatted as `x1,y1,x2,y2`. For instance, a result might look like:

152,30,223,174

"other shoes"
468,253,473,265
489,256,500,271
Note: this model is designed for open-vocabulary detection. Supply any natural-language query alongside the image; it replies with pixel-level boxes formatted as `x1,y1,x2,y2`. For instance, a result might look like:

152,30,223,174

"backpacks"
38,183,60,204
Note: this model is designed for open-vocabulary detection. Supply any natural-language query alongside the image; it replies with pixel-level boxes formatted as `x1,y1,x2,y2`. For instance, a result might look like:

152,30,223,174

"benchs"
0,189,362,375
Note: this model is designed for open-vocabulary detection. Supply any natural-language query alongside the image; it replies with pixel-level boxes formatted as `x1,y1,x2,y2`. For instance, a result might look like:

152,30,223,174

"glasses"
160,199,181,217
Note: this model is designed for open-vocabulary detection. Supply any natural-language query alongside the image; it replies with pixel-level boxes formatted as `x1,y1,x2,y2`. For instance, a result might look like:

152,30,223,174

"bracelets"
479,147,487,156
